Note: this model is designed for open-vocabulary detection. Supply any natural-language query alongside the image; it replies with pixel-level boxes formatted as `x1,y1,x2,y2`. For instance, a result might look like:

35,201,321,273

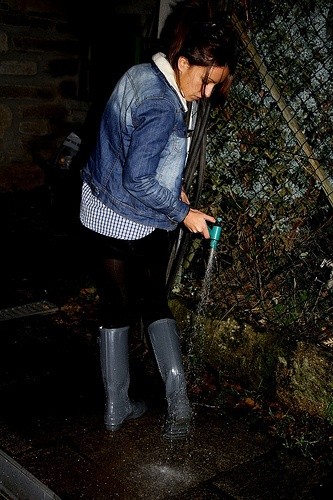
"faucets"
210,218,222,250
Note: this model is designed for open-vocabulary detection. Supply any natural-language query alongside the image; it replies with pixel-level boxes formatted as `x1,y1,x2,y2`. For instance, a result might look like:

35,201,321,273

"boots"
147,318,192,441
99,326,147,431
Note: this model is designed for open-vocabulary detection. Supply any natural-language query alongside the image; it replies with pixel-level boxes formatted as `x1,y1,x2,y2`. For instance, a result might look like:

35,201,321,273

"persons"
79,13,240,438
